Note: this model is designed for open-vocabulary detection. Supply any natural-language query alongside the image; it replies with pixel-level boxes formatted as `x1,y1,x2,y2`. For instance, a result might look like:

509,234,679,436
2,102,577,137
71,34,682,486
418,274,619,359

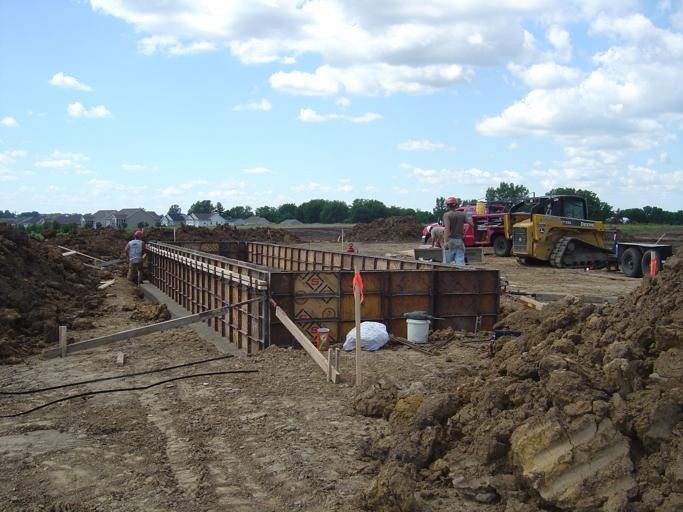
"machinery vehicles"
504,191,620,270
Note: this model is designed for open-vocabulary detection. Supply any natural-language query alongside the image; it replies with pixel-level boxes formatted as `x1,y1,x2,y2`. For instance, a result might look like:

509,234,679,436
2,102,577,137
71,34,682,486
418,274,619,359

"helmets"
135,231,143,238
447,197,457,206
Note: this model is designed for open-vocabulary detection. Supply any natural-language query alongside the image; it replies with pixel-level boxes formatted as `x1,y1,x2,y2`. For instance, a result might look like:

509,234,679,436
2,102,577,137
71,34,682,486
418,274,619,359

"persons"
347,243,354,252
427,226,445,248
443,197,474,265
125,228,149,284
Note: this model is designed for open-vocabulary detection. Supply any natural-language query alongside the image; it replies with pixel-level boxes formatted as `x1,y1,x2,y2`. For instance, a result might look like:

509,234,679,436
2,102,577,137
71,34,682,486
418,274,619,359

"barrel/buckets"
317,328,330,350
404,318,431,343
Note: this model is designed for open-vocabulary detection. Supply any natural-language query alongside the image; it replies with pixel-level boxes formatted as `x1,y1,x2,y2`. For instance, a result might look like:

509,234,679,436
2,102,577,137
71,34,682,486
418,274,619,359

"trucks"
418,201,518,254
